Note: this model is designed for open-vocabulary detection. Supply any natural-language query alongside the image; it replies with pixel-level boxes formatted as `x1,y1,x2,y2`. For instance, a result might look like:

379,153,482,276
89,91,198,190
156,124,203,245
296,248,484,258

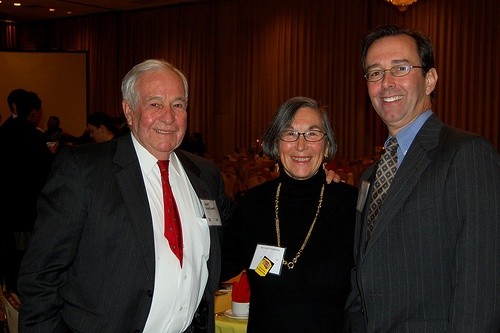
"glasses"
278,130,326,142
365,64,428,82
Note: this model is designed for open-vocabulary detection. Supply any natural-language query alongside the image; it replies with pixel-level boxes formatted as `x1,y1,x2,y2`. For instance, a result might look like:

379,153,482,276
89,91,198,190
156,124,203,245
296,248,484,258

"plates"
224,309,249,319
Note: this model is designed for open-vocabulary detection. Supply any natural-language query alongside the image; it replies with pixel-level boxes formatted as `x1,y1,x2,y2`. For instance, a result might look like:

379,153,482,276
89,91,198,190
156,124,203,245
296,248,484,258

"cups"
232,301,250,316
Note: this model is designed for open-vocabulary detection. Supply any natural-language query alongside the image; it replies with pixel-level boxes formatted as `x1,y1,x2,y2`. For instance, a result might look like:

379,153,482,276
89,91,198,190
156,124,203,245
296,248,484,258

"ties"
156,160,184,269
367,138,399,249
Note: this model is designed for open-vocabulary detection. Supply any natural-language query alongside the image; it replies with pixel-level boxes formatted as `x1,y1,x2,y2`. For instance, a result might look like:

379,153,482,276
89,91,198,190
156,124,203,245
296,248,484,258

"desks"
215,313,249,333
